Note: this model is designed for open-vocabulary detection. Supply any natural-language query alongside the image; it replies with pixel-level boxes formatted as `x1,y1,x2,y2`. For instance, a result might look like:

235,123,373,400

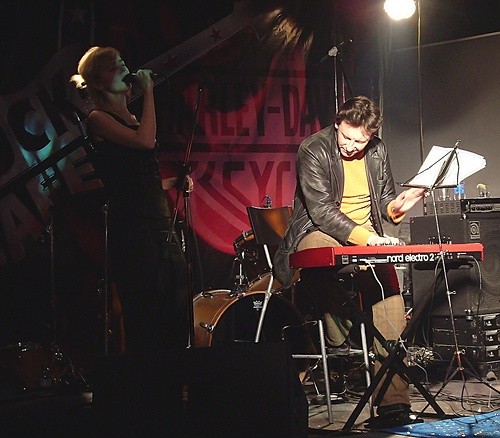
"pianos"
289,237,485,436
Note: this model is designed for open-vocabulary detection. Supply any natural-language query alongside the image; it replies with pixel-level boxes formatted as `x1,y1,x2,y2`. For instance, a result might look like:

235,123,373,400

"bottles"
454,180,466,200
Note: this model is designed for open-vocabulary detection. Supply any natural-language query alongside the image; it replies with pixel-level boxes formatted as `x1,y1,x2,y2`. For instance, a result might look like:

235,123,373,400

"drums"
233,230,284,293
191,288,307,375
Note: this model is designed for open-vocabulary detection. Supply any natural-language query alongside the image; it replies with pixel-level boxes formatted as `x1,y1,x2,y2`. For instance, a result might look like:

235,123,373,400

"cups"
438,188,449,201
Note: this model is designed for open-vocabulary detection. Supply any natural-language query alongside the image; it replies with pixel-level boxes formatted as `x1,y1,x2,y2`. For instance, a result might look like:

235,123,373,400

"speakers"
411,212,500,318
156,340,308,438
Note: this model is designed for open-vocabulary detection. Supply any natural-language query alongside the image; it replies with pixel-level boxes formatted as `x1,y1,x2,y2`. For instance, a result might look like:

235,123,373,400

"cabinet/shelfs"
432,312,500,378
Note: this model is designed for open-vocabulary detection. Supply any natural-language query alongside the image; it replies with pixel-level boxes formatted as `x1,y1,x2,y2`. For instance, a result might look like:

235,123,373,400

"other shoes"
328,337,351,354
377,409,424,425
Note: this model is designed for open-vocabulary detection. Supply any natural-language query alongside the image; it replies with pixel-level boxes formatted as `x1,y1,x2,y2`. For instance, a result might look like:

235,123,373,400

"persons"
275,95,425,424
70,46,194,438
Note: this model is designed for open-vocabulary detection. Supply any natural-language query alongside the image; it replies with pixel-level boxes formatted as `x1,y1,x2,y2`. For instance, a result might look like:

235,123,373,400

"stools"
247,206,374,425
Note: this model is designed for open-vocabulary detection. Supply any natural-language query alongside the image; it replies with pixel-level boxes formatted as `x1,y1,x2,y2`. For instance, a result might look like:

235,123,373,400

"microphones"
124,72,159,82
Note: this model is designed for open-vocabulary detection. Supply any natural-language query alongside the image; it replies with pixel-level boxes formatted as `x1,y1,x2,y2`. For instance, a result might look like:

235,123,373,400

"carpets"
374,410,500,438
